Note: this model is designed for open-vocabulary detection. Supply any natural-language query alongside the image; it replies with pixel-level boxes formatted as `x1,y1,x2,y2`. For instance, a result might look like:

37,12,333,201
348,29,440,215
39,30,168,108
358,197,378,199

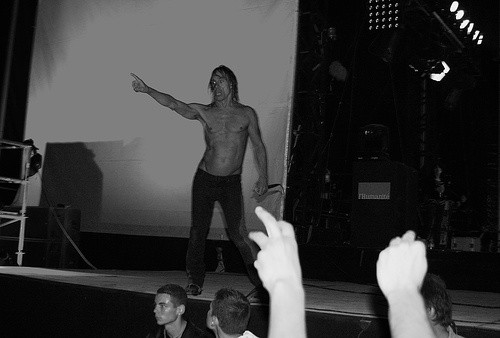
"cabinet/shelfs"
0,138,34,265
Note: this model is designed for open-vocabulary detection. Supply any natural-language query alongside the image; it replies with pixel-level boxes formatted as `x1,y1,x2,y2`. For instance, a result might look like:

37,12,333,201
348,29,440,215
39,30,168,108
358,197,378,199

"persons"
206,287,260,338
248,206,439,338
129,65,270,300
145,285,207,338
420,273,463,338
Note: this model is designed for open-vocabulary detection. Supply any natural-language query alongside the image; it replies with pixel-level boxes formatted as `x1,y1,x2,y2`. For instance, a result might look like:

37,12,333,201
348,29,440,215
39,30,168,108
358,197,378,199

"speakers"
46,207,81,270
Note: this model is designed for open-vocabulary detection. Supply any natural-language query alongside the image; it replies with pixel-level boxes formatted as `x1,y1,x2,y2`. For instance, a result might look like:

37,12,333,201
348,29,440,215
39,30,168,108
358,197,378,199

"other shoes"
185,283,203,296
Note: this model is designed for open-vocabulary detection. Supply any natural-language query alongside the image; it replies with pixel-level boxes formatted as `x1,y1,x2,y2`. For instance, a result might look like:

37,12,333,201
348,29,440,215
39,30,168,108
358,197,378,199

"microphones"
254,184,280,192
